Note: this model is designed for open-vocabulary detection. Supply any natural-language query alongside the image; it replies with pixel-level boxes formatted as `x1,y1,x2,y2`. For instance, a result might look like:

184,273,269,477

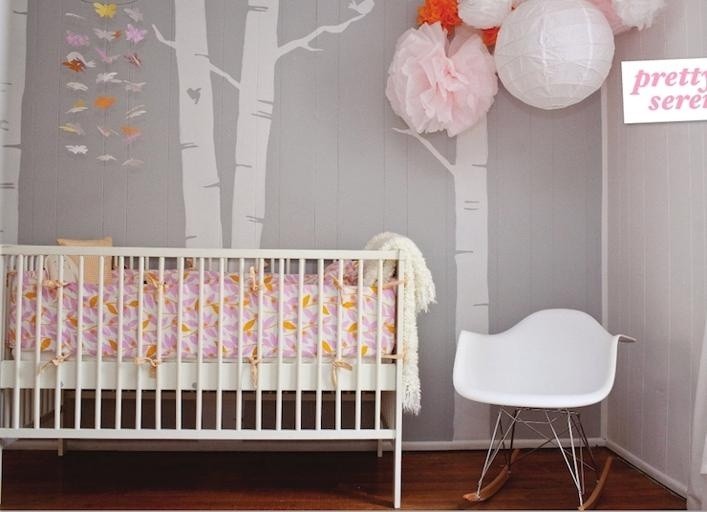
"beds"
2,245,405,508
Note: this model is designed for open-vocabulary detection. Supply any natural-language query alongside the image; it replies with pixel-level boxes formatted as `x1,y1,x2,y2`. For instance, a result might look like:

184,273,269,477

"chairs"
452,307,636,512
